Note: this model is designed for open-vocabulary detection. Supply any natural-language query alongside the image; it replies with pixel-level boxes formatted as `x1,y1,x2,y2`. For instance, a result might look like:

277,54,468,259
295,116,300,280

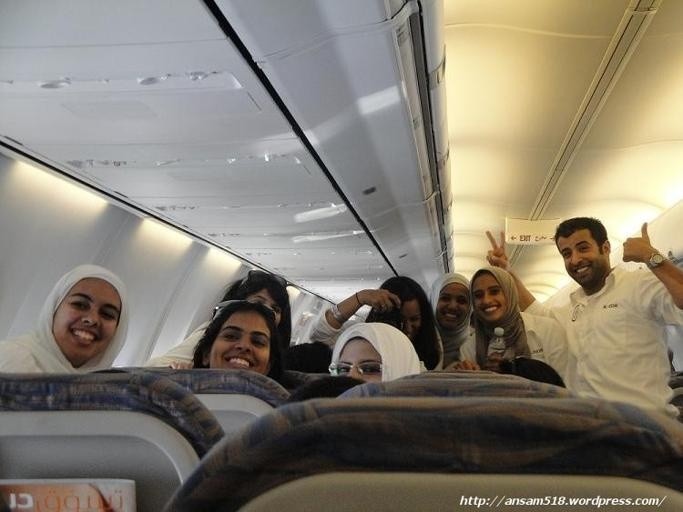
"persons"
146,266,565,403
0,263,130,374
485,221,682,419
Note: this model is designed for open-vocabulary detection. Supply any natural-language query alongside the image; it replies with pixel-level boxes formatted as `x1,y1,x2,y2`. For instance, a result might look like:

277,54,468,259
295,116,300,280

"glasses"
329,363,382,375
240,270,287,288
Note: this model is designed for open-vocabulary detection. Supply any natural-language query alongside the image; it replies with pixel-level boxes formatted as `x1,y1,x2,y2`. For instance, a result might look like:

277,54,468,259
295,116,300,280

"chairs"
1,370,683,512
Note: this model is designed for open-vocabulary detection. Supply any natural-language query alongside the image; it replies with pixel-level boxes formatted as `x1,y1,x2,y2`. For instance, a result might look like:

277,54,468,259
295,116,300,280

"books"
0,478,136,512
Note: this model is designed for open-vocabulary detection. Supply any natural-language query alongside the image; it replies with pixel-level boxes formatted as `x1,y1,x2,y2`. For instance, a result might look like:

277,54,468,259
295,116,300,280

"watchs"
645,251,666,270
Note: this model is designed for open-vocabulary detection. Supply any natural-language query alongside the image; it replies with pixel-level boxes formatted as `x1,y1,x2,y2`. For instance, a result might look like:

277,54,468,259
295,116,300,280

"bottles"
487,327,506,359
